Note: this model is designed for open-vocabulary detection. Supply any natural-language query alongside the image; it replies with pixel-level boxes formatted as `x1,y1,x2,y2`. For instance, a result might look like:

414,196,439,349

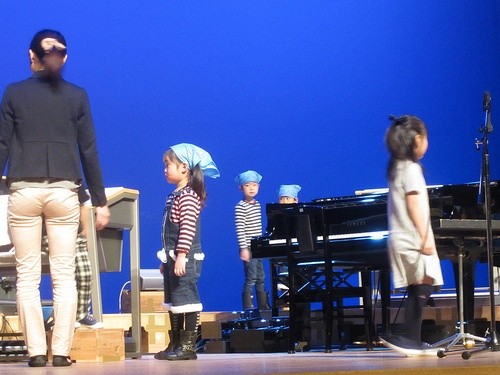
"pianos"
251,181,500,355
0,185,142,361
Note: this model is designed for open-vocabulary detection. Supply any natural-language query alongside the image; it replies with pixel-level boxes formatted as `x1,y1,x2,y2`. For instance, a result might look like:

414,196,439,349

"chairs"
283,204,374,354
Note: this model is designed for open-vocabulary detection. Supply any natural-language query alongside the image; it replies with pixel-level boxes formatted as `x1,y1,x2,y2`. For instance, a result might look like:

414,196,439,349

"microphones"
484,91,493,131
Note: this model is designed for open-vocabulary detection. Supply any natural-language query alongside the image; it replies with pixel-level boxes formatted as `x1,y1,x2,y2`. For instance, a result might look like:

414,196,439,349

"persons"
272,185,301,311
42,217,104,330
380,115,444,358
0,29,111,367
235,170,272,310
154,143,220,361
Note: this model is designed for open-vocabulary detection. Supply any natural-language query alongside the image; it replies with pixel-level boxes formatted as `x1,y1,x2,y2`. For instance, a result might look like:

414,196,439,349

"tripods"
430,236,489,359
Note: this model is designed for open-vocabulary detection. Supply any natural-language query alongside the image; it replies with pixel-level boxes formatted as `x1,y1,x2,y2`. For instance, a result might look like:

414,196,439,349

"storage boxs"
121,290,171,314
47,328,125,364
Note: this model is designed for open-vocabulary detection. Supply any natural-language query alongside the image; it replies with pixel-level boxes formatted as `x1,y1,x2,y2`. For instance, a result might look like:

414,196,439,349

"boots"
256,291,273,312
242,292,258,310
154,330,180,360
165,329,199,360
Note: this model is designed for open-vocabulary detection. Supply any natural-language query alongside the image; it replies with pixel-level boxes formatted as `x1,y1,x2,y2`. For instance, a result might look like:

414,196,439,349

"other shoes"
75,314,104,329
29,355,46,366
377,334,446,355
53,355,72,366
